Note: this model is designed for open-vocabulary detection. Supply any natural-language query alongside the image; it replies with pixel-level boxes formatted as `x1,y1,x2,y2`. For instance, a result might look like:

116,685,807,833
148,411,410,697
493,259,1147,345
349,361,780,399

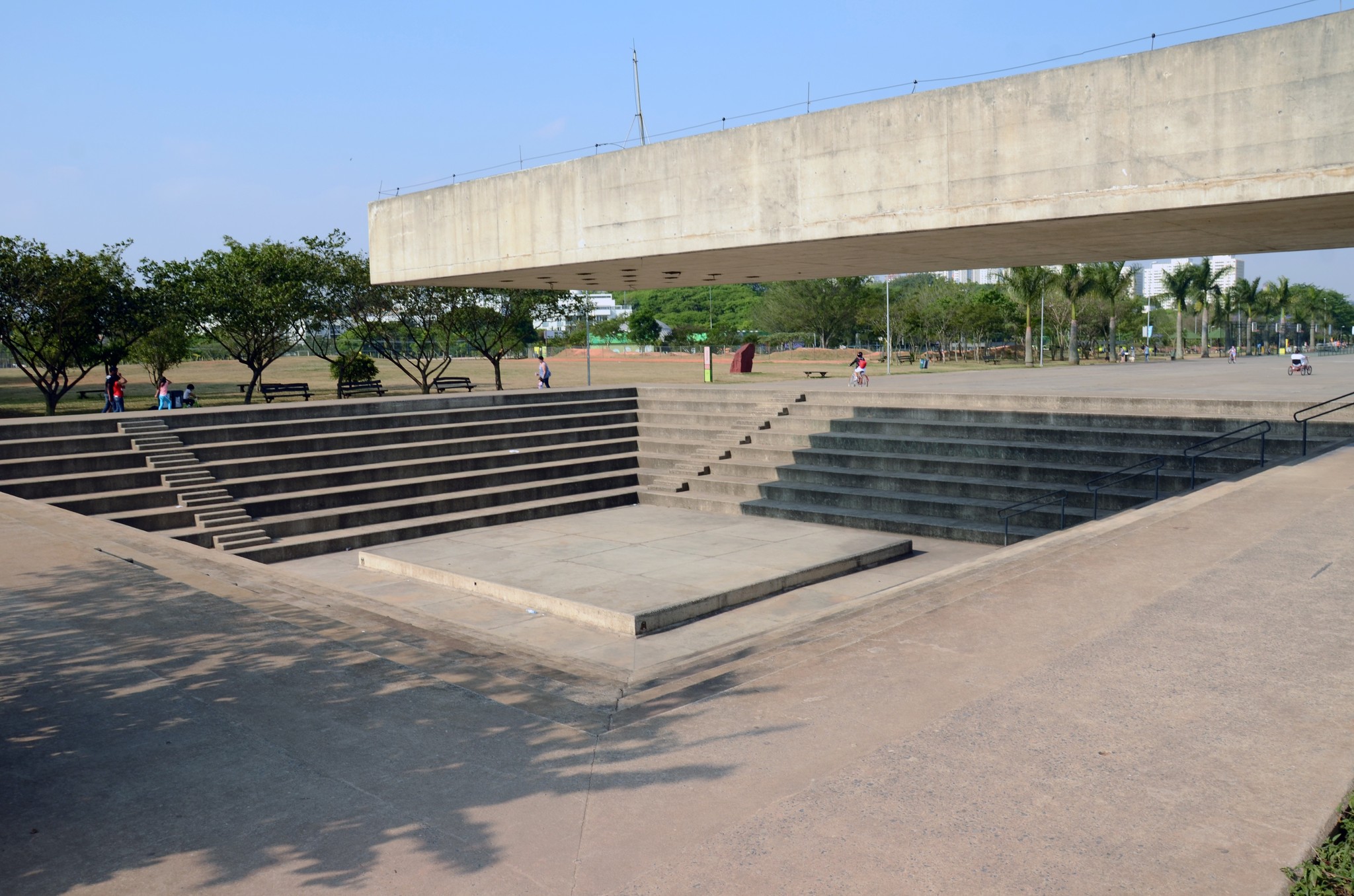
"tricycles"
1288,356,1312,376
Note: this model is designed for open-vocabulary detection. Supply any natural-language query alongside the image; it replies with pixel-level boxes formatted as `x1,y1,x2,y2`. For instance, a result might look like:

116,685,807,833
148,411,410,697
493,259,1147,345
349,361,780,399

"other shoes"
853,378,858,381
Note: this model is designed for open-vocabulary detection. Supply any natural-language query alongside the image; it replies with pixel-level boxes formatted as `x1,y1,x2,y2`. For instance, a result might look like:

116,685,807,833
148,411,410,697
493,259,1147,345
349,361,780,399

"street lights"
1339,325,1345,350
1323,298,1327,351
1147,261,1158,347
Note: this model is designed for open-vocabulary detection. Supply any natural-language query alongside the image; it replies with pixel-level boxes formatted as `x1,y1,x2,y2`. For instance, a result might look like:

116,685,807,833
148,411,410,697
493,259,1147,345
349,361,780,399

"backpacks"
857,357,867,369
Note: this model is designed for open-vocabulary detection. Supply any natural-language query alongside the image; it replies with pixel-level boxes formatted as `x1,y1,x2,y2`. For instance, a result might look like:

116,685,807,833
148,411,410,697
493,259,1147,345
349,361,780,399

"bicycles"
848,364,869,387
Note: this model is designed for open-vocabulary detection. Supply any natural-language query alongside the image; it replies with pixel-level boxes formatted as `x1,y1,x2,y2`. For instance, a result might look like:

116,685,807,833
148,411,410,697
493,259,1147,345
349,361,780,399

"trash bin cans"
919,358,929,369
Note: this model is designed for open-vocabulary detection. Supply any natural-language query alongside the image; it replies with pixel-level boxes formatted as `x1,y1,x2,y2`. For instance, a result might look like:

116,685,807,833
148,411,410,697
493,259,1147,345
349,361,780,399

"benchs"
924,353,941,362
236,382,316,401
336,379,390,399
75,389,106,400
432,376,478,394
804,370,828,378
983,355,1000,366
898,355,913,365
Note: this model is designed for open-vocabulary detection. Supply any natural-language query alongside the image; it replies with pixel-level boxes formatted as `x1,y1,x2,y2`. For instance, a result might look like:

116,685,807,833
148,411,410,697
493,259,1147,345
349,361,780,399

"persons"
183,384,198,408
848,352,866,384
1101,340,1348,369
535,356,551,388
102,367,128,413
538,377,543,388
155,376,172,410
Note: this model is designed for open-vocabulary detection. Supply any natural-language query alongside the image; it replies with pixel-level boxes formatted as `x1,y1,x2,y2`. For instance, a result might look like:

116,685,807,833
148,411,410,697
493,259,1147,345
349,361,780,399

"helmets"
857,352,863,358
